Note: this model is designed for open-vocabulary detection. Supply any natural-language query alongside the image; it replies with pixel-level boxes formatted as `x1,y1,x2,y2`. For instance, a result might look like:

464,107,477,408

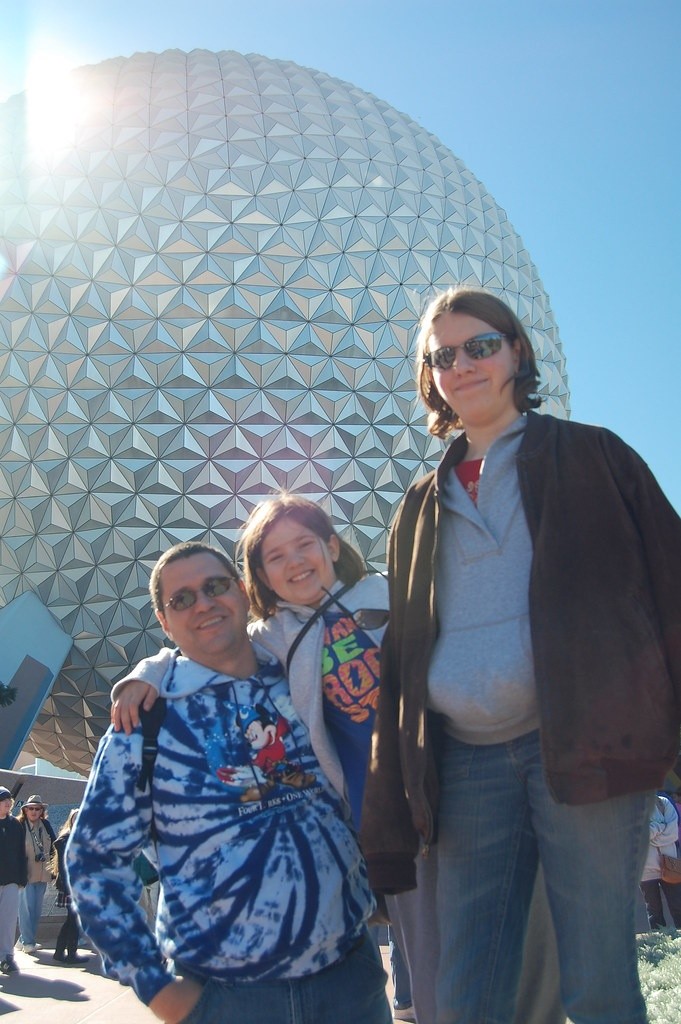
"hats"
0,786,11,799
20,795,49,810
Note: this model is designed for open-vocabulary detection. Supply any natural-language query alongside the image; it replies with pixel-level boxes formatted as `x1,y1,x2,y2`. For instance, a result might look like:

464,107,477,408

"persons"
110,498,419,1023
62,540,396,1023
390,286,679,1024
0,768,681,1023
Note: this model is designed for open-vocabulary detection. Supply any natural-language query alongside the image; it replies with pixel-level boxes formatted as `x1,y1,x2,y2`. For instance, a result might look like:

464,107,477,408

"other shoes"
392,1006,415,1022
0,959,19,975
53,951,67,960
64,951,89,963
22,944,36,953
15,940,42,951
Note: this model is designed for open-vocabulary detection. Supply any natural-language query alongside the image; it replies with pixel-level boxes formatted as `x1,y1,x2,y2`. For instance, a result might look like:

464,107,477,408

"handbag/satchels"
660,854,681,883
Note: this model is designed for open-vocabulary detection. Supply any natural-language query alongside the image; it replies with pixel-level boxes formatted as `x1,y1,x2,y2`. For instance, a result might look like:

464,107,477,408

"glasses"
162,577,236,611
27,807,41,811
321,561,391,630
423,332,506,371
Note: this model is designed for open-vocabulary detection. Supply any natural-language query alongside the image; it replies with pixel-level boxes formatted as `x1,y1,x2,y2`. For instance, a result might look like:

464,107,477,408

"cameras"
34,853,46,862
